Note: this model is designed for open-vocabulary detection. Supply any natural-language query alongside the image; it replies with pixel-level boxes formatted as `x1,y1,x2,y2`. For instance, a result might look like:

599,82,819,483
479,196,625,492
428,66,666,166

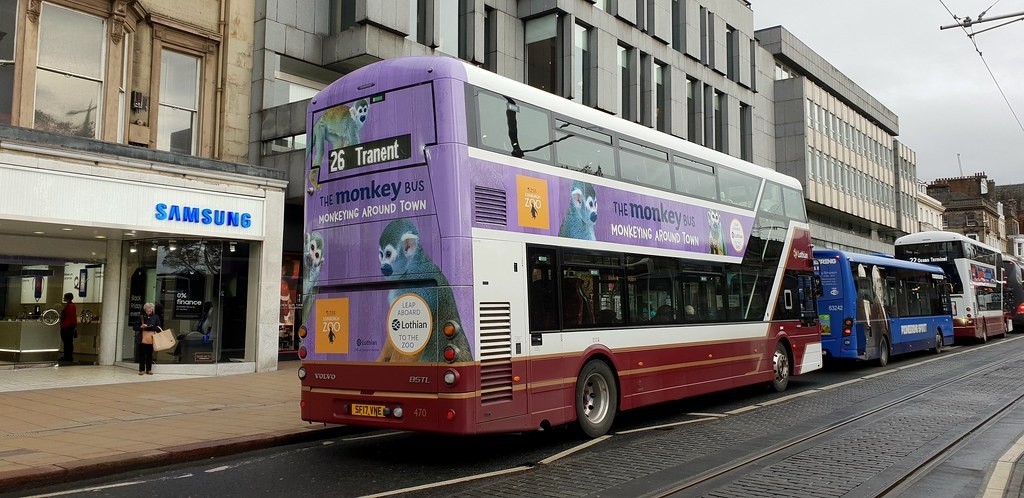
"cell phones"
34,276,41,299
78,268,87,298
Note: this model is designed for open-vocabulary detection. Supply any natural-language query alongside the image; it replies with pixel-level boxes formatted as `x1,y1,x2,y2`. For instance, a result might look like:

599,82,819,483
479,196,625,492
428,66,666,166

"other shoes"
57,356,73,362
138,370,144,375
146,370,153,375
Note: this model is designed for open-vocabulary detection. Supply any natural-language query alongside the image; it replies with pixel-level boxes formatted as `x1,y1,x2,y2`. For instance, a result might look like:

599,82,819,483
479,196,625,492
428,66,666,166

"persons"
59,292,78,362
166,301,214,356
137,302,162,375
644,303,783,325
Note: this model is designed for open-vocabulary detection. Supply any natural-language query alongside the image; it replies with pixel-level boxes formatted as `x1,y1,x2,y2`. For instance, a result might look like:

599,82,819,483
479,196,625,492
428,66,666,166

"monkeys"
558,180,598,241
378,219,473,362
707,209,727,255
302,231,324,325
306,99,372,191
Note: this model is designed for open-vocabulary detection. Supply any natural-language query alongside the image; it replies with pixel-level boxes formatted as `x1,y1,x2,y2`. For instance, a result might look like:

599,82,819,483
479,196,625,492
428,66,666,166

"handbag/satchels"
74,328,78,339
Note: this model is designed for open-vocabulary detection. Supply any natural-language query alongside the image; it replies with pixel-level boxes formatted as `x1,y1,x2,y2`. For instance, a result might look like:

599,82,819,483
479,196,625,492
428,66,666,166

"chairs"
181,307,215,363
532,277,596,328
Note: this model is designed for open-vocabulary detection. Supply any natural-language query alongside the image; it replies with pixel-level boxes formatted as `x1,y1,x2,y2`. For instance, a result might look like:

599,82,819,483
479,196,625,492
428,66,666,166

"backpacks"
140,314,157,345
153,325,175,350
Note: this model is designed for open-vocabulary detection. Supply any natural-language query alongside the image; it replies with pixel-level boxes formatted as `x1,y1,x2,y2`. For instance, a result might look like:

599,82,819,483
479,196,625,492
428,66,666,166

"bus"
810,247,958,368
1000,258,1024,330
295,56,823,442
890,233,1015,340
